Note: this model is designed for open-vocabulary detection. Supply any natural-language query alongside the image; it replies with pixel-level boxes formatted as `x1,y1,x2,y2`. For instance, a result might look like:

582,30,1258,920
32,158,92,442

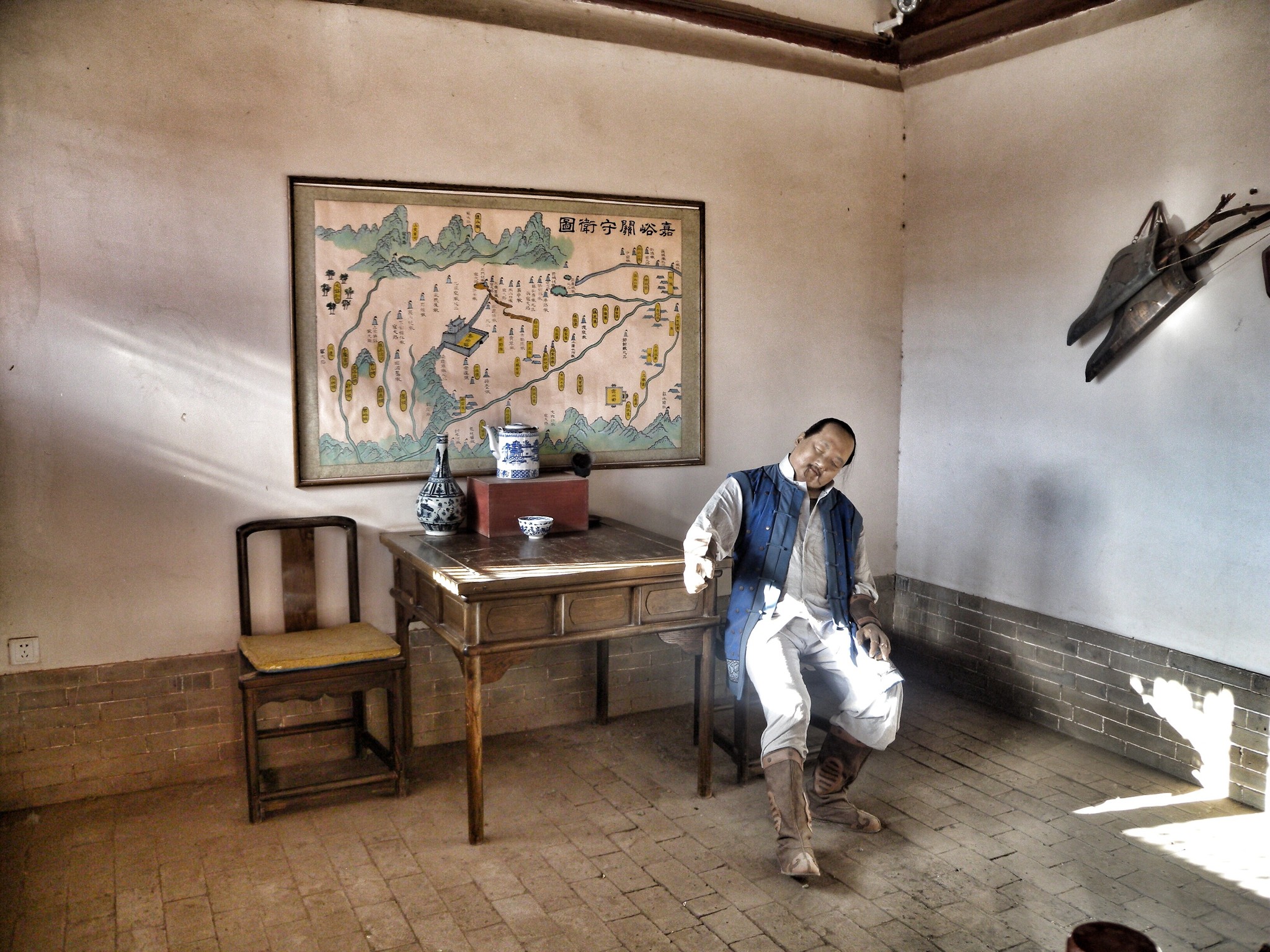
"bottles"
415,433,469,536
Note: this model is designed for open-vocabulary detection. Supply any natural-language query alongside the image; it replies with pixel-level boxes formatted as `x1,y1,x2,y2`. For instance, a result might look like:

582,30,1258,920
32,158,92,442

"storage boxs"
463,472,594,539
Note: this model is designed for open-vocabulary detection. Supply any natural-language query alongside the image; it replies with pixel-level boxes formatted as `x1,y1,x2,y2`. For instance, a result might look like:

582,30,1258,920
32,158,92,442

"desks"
376,512,736,848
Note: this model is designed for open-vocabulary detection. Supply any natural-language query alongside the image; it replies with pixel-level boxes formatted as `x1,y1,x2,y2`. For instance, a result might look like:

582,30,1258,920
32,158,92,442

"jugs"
482,422,540,480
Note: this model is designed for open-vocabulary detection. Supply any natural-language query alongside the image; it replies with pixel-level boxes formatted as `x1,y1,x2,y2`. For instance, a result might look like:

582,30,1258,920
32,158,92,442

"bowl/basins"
518,516,554,539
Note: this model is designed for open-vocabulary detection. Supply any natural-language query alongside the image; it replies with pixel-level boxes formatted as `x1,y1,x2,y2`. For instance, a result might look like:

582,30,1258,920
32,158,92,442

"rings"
880,643,888,648
871,640,879,644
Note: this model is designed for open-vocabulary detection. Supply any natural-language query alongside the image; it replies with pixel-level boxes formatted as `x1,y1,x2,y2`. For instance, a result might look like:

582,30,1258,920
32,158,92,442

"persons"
682,418,906,878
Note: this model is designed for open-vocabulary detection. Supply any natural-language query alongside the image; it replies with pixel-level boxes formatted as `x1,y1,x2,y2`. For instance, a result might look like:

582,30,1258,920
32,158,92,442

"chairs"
689,591,836,794
227,515,412,831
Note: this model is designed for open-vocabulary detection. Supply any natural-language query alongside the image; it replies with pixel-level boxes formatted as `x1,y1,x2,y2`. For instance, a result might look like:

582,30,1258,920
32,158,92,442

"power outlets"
4,634,44,670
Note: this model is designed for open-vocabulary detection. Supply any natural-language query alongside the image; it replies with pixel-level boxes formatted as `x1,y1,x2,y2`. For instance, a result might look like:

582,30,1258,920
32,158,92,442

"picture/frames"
280,173,713,495
